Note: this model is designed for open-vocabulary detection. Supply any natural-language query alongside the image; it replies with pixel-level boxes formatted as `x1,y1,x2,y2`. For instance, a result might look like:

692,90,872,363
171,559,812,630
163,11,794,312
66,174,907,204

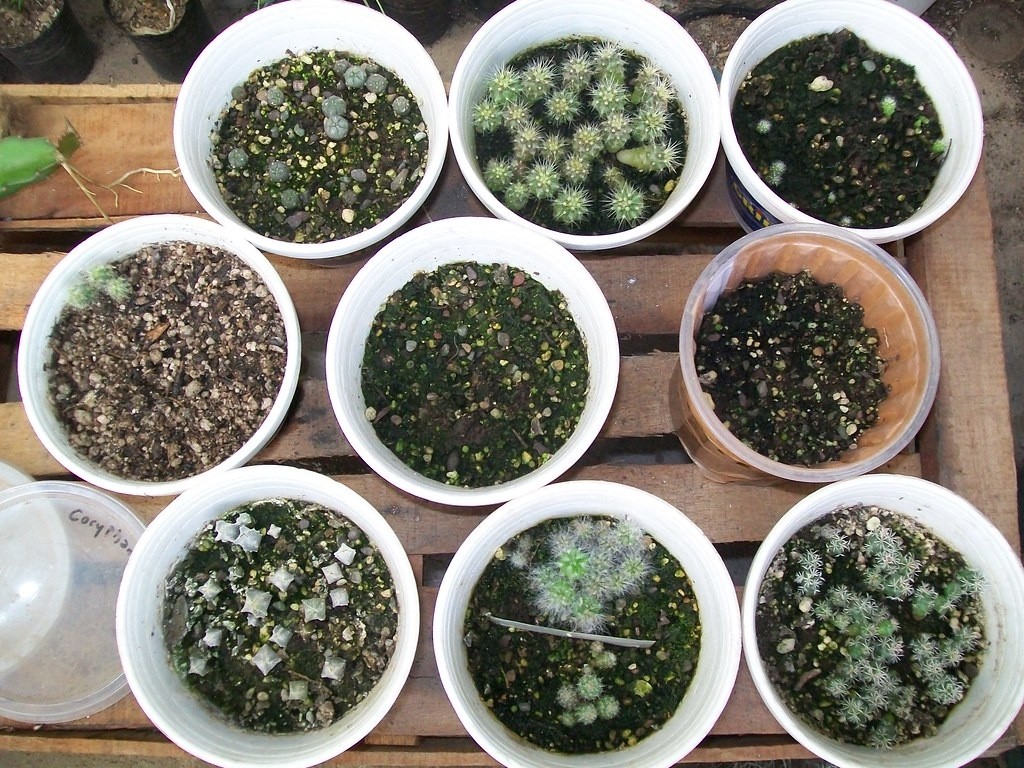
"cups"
0,481,148,724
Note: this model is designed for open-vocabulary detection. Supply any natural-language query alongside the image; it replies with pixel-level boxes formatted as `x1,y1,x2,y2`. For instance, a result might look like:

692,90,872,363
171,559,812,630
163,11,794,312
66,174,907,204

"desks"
2,82,1024,767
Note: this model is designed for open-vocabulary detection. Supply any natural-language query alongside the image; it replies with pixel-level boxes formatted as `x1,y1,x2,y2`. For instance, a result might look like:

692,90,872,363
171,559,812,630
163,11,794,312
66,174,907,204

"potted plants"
718,0,985,247
740,474,1024,766
442,0,724,251
325,216,622,509
171,0,450,261
19,211,302,500
113,463,419,766
433,480,739,767
671,222,942,488
